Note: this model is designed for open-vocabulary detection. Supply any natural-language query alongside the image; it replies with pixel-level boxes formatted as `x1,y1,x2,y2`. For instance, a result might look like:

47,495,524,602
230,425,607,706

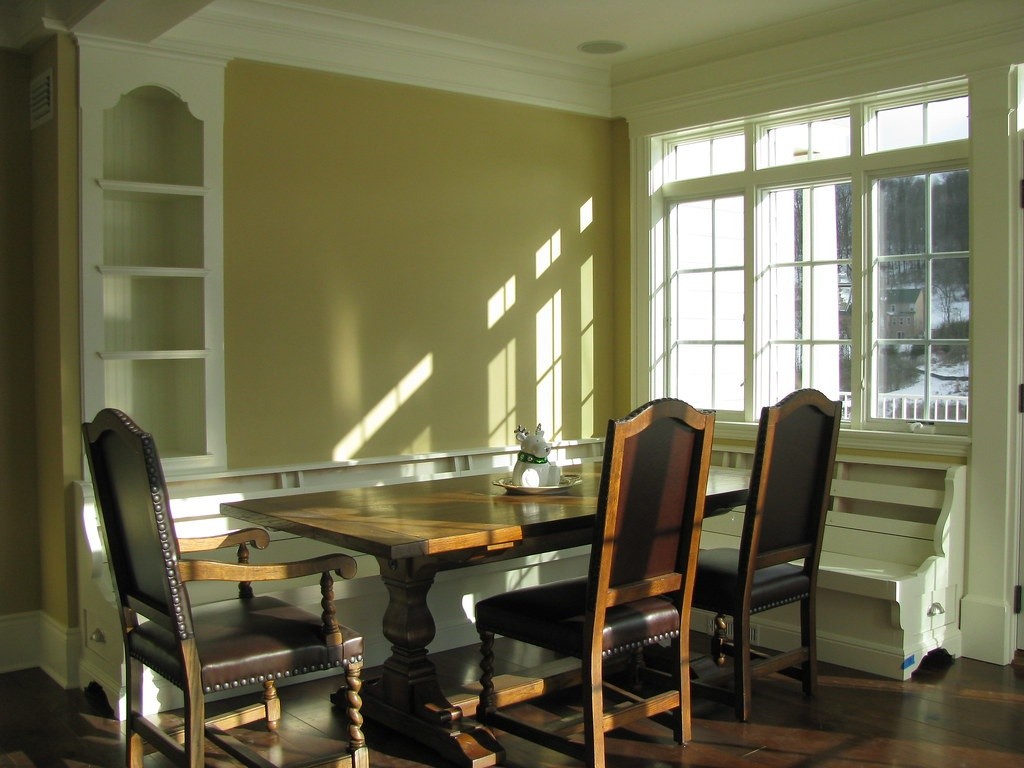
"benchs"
685,444,970,682
72,437,607,723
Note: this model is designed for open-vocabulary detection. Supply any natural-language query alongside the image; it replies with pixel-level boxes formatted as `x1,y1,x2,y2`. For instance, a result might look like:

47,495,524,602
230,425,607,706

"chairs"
81,407,369,768
624,388,845,723
475,397,716,768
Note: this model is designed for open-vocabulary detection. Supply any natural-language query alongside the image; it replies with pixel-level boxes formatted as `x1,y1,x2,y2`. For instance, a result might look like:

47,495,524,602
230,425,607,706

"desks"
218,460,753,768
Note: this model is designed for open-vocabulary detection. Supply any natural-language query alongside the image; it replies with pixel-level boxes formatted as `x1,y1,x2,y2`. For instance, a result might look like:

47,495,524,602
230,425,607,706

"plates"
492,476,582,495
